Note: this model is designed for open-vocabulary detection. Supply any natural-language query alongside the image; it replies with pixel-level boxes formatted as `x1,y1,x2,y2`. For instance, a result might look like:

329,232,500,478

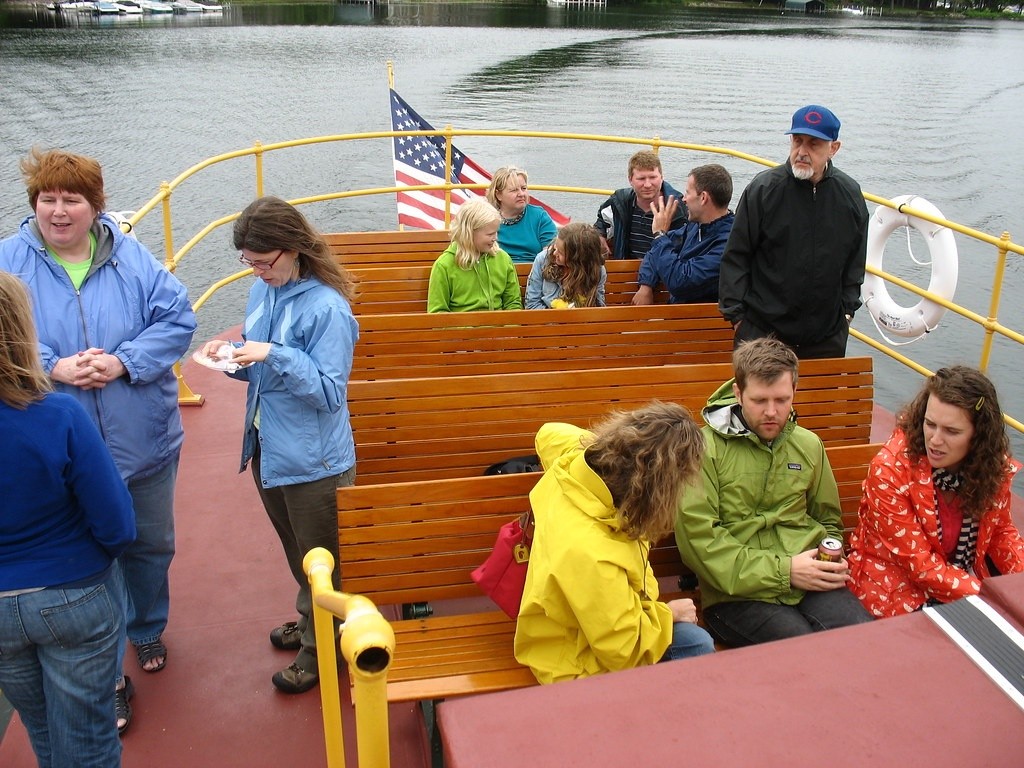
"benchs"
345,260,672,317
334,445,886,709
319,230,453,269
348,302,736,380
345,352,874,487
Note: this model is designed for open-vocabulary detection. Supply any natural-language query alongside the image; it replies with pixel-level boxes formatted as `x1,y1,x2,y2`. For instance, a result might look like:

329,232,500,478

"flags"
387,88,572,235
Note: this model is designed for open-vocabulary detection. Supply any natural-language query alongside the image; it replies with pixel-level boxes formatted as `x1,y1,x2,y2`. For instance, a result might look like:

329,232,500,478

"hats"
783,105,840,143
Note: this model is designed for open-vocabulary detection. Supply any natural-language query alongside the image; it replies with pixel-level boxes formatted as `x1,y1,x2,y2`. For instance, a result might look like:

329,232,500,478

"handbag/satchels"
472,508,535,620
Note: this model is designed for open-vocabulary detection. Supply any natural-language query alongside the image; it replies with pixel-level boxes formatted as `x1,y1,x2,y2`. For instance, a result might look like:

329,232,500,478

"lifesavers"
859,193,959,339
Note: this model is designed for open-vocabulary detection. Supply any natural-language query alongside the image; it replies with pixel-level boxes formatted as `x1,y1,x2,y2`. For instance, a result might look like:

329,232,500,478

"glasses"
238,249,286,270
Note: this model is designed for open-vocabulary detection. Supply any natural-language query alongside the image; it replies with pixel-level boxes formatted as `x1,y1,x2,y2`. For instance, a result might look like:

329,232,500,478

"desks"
435,570,1024,767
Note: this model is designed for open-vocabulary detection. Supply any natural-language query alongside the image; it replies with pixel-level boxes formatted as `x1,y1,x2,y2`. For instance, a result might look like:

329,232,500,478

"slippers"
136,640,167,671
114,675,132,734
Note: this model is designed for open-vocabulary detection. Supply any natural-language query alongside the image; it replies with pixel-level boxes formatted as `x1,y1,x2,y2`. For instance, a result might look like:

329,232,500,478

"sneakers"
269,621,303,650
271,659,319,694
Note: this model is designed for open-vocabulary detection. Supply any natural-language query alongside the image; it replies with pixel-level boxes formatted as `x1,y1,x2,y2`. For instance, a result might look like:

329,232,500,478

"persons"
1,150,199,738
522,222,607,309
590,151,687,261
843,365,1023,622
427,197,522,313
714,105,870,362
485,166,559,263
231,194,359,694
631,164,735,307
507,398,716,689
673,339,874,647
2,273,139,768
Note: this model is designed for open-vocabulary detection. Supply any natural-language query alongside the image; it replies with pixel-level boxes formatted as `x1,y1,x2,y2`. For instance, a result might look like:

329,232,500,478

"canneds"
816,537,843,573
